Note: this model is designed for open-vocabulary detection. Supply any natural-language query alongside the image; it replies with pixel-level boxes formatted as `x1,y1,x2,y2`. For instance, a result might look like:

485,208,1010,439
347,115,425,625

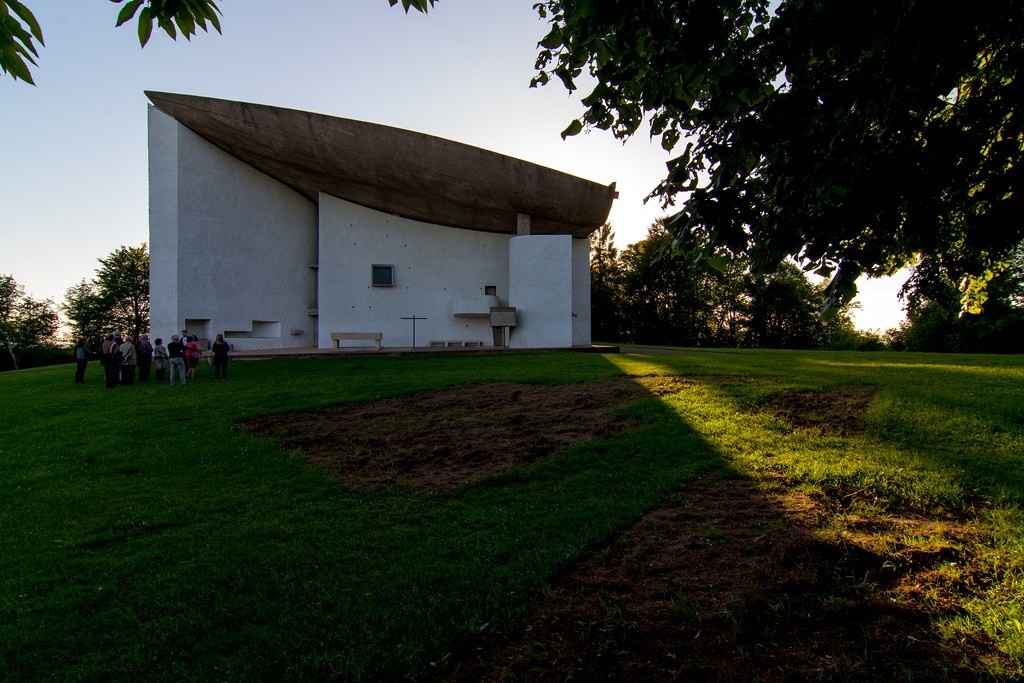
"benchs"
330,332,382,350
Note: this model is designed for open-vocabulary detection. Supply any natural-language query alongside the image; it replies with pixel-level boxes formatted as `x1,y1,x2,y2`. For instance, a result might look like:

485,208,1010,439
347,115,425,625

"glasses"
115,334,120,336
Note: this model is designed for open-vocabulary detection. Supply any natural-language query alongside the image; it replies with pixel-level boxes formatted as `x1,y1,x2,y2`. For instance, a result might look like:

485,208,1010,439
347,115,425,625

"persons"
100,330,202,390
73,338,88,383
212,334,230,382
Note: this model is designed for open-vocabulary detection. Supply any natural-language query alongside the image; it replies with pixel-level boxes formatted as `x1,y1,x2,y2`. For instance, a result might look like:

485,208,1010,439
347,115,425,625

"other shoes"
170,384,176,386
182,384,188,385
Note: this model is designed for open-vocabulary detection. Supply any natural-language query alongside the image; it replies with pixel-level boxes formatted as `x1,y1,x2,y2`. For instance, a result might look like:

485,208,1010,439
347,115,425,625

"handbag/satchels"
157,348,165,354
192,352,202,359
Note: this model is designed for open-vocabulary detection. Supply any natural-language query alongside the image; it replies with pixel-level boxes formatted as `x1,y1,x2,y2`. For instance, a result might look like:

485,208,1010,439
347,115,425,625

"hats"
125,337,133,341
